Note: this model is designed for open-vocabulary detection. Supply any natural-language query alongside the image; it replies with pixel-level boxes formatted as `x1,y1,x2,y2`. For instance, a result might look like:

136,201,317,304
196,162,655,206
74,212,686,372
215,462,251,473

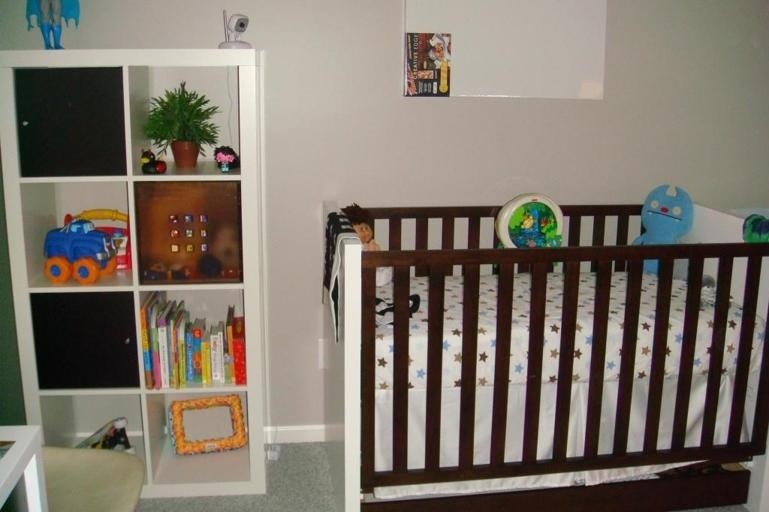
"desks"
1,425,51,510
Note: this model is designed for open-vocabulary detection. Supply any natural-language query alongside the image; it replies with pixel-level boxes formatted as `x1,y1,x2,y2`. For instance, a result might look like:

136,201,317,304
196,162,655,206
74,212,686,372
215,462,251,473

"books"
139,293,245,389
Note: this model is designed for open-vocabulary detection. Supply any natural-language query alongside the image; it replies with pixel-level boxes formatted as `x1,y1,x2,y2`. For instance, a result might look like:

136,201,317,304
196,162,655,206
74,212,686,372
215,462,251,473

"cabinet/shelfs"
1,48,269,500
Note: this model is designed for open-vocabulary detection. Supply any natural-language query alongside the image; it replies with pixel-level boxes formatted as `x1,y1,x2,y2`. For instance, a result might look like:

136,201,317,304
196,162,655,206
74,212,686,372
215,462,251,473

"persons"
427,37,450,74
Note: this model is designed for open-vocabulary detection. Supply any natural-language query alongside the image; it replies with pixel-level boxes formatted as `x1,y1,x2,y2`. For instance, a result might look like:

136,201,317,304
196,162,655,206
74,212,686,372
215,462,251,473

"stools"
14,441,147,510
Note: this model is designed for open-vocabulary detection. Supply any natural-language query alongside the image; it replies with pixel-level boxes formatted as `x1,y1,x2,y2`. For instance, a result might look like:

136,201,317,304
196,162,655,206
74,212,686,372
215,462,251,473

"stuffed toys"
340,205,382,251
630,181,693,279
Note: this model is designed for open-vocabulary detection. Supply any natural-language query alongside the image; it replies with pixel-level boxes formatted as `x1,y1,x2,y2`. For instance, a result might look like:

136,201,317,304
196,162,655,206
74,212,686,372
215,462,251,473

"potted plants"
141,80,222,169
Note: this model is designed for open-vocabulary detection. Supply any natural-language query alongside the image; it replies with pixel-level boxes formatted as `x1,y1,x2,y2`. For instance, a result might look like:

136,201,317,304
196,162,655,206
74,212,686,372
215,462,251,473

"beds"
320,196,769,512
